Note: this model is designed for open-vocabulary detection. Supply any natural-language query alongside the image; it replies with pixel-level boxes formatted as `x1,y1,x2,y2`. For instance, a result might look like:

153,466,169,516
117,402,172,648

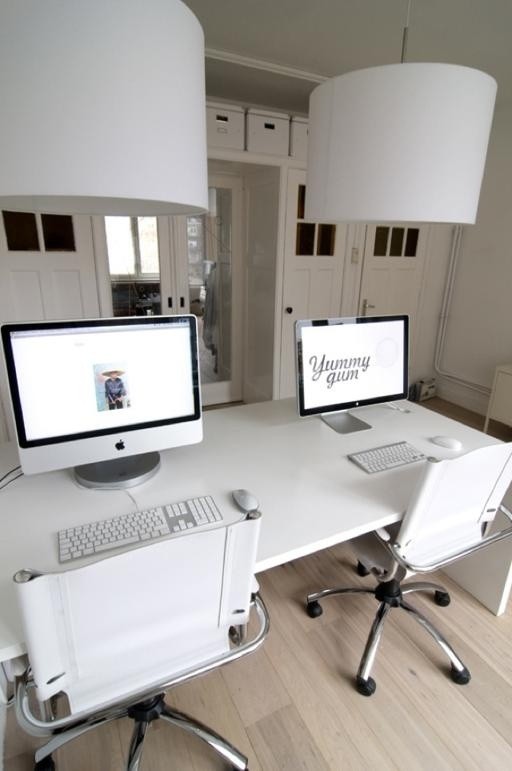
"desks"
1,393,506,679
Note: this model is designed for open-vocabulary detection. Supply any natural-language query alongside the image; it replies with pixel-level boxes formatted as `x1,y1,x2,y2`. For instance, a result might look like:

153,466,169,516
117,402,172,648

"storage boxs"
205,96,306,161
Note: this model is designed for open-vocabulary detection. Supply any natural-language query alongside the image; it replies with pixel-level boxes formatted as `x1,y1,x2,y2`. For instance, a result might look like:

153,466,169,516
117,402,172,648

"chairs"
306,442,512,698
15,507,268,770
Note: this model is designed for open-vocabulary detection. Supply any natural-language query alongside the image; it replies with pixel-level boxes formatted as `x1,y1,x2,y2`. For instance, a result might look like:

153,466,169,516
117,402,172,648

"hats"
102,369,124,377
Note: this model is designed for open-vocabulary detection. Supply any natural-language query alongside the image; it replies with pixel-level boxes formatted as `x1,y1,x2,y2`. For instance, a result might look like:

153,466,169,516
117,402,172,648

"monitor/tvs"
0,312,205,491
293,313,410,435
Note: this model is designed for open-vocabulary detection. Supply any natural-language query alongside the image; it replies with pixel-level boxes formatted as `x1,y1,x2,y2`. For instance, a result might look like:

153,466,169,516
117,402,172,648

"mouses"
431,436,462,450
232,488,260,512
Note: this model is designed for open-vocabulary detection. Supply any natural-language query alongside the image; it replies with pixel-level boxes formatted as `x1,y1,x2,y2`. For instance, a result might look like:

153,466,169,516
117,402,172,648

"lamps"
0,0,216,221
293,3,501,226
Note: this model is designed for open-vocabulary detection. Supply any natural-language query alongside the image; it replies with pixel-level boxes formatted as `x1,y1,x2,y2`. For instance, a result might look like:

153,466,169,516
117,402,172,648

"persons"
102,370,127,410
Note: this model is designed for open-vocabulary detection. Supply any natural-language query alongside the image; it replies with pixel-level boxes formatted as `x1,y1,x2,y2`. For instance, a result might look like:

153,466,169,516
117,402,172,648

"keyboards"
346,440,428,474
57,494,223,565
